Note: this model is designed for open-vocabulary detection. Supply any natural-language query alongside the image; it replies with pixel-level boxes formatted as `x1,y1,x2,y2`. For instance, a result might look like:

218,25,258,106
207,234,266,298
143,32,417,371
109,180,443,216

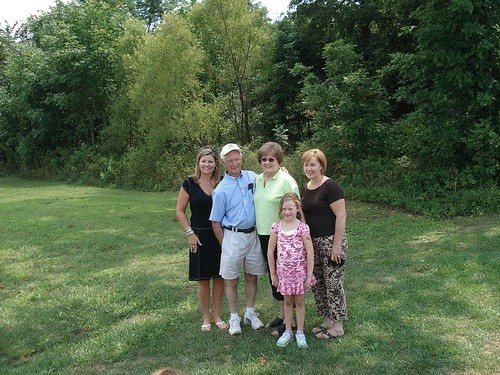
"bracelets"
184,227,194,236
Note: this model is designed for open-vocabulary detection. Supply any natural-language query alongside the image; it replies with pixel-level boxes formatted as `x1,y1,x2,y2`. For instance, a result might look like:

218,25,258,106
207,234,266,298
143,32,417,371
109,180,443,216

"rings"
338,255,341,258
192,246,195,248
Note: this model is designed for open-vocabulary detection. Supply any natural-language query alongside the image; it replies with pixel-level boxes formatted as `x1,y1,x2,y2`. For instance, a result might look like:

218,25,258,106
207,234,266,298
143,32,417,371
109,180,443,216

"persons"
209,143,289,335
177,145,224,331
299,149,347,339
267,192,317,349
253,142,301,337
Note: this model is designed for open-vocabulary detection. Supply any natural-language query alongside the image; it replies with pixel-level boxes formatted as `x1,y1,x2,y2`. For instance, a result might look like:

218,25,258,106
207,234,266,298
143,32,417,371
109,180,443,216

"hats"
221,144,242,159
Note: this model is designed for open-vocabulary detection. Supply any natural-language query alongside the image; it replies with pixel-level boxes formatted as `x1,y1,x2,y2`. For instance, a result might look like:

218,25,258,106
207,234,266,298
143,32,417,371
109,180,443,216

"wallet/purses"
328,255,345,268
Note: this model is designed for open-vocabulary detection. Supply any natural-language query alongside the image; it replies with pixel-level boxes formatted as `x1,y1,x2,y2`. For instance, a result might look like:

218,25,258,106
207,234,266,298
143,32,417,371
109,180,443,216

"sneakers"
294,332,308,349
228,316,242,335
244,312,264,329
276,331,295,347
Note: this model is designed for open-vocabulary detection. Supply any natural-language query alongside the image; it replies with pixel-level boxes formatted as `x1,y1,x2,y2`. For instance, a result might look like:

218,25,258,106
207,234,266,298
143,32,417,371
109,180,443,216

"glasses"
261,157,276,162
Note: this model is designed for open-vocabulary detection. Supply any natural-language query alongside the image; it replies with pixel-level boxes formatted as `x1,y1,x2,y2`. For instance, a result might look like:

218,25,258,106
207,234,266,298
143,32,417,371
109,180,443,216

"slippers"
212,318,228,330
265,315,283,328
270,323,296,337
311,324,345,339
201,320,212,332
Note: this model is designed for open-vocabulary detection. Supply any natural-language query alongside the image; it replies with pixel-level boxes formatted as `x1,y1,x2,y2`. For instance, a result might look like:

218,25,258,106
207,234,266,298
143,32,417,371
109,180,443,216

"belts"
223,226,255,234
